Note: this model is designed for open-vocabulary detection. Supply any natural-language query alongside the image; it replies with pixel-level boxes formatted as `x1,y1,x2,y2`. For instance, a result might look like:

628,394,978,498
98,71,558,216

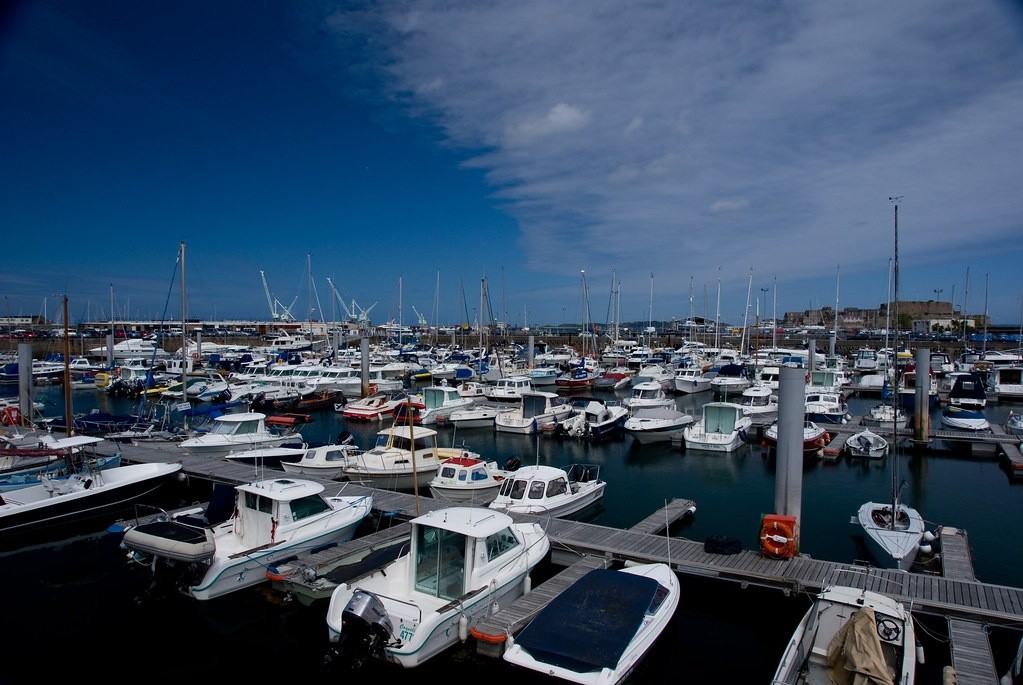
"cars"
186,328,261,337
0,329,172,339
262,329,313,336
677,324,1023,342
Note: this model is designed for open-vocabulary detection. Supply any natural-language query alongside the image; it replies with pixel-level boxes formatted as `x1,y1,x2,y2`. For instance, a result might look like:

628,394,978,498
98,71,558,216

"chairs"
51,480,65,496
42,478,53,498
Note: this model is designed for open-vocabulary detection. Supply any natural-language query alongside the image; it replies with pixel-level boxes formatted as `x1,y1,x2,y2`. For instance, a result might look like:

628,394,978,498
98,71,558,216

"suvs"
329,328,346,336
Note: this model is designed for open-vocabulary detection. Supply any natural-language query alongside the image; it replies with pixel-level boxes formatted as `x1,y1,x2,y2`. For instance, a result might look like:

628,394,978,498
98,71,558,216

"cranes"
327,278,358,322
260,271,279,321
410,306,427,327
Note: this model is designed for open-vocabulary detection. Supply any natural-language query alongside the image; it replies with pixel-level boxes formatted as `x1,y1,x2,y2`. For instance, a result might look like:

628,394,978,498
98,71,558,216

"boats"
324,504,553,668
858,501,924,570
108,475,376,602
429,455,516,506
0,339,1023,471
504,564,681,685
0,460,181,551
280,445,359,480
490,461,607,518
341,426,481,490
771,566,917,684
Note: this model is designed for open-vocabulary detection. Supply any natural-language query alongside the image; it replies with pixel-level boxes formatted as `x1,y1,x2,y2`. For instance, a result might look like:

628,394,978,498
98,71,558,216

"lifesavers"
805,375,811,384
760,522,793,558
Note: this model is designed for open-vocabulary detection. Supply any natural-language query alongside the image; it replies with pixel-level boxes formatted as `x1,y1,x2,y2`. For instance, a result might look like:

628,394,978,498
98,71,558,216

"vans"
171,328,183,337
